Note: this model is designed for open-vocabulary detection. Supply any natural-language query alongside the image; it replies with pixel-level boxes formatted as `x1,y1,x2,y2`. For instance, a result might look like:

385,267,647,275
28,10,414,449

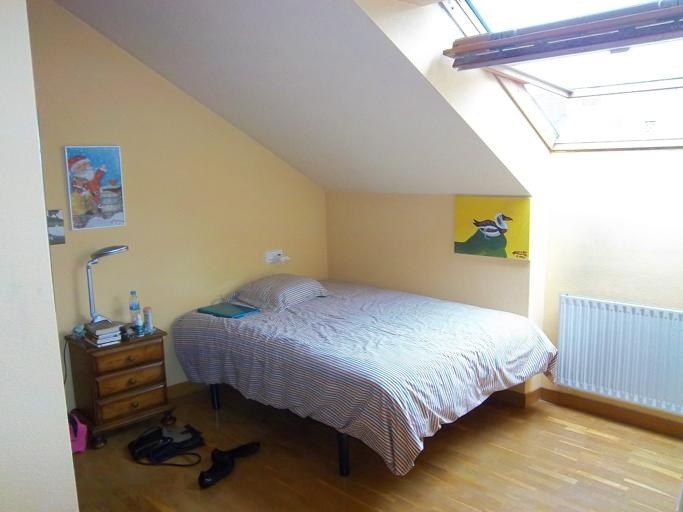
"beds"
172,280,560,477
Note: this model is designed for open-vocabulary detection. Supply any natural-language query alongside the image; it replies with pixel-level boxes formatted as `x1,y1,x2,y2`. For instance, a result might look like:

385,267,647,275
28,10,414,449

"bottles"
128,290,140,323
134,314,144,338
143,307,153,333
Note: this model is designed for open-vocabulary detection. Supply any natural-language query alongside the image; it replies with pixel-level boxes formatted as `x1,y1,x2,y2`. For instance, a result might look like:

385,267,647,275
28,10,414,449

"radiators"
558,292,682,419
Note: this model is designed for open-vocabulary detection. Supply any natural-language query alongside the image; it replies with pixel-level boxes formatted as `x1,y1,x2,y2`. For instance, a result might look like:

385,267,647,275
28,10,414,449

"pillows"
224,275,328,313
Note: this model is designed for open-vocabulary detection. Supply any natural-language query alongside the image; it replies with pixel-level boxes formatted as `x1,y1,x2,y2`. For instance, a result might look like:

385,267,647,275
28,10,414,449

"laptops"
200,303,259,319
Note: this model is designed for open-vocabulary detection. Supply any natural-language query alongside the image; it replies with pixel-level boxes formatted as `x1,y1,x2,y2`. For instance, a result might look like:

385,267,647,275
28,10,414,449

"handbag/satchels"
128,425,205,464
68,407,88,454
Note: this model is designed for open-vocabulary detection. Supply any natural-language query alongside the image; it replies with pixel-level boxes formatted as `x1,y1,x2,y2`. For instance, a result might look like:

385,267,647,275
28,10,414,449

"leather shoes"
211,442,260,465
199,454,234,489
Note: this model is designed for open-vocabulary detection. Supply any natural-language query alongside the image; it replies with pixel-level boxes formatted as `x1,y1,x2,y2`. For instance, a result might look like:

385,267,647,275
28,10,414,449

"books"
197,302,260,318
83,320,122,348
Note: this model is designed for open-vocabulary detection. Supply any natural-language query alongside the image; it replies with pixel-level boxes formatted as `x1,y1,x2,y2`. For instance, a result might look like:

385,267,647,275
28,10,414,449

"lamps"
85,244,129,324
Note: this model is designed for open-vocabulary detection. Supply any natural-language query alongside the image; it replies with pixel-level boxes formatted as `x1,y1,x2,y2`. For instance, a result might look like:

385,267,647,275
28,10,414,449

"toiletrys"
143,306,154,333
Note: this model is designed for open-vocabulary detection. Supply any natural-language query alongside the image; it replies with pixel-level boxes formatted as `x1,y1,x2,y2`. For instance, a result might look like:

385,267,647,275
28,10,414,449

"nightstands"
67,328,176,448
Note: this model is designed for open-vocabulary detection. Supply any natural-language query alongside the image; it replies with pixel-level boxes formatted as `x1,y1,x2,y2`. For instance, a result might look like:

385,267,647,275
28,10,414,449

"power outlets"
266,249,284,265
